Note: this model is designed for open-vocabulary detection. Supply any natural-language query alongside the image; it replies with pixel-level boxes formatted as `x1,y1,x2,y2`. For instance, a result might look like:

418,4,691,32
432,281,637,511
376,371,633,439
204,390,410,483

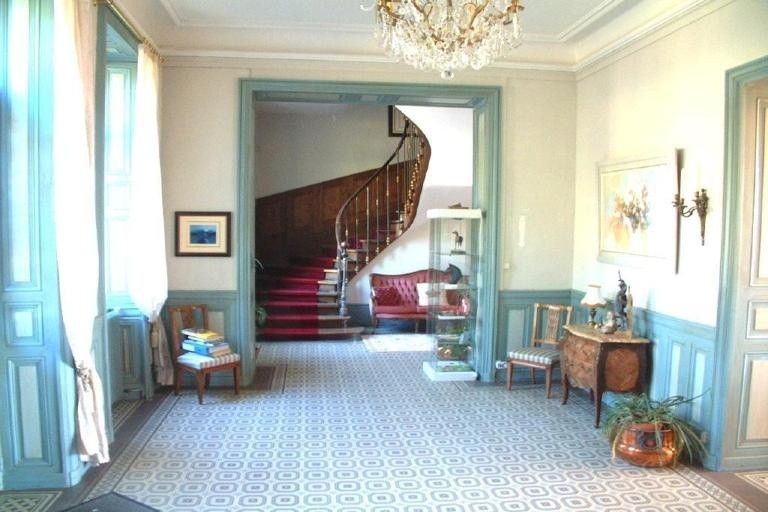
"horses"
444,263,462,284
452,230,463,250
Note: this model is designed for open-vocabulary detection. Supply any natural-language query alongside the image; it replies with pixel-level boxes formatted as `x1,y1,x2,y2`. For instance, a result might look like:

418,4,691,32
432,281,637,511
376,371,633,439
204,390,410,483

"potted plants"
601,384,715,474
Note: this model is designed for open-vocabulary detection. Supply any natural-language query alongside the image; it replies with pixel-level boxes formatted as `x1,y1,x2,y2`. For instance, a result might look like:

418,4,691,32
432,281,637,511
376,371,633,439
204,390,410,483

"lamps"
361,0,527,80
579,284,608,328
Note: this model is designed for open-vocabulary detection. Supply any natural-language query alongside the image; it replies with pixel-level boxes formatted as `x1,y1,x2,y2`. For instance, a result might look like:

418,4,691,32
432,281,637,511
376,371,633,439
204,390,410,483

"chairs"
506,302,574,399
163,302,241,405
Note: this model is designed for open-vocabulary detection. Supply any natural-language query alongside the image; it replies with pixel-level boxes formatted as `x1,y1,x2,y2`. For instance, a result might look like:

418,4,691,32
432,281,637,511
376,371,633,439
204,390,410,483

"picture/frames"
387,104,420,138
174,210,231,256
595,148,684,275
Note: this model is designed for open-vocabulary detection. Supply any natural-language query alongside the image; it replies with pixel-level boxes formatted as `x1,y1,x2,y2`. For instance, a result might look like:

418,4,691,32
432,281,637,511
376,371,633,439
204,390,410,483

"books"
180,328,232,358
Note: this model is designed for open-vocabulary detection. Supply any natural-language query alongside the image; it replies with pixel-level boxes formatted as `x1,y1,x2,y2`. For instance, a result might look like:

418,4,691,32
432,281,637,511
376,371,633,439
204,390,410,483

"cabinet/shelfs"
422,209,483,383
557,324,651,428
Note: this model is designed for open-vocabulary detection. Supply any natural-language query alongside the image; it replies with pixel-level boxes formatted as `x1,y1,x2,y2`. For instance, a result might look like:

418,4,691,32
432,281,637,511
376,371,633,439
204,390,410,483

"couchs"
368,268,470,335
